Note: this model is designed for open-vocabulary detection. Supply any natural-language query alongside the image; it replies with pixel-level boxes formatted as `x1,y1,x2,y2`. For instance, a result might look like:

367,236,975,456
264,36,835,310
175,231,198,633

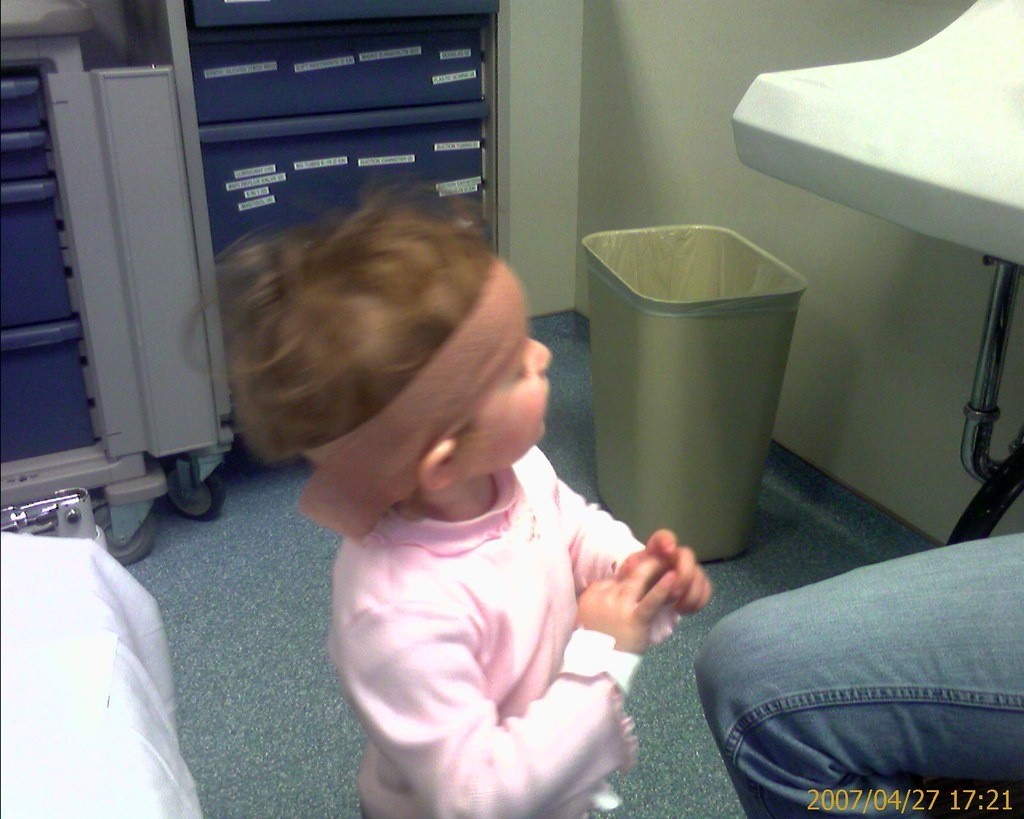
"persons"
203,204,711,819
697,534,1023,819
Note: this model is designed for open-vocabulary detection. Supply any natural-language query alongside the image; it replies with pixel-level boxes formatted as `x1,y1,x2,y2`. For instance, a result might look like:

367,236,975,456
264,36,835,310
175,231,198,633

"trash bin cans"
578,225,803,561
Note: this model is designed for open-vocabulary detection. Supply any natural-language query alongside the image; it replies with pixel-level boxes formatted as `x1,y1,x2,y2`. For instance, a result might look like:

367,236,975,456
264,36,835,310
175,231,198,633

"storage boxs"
0,0,494,464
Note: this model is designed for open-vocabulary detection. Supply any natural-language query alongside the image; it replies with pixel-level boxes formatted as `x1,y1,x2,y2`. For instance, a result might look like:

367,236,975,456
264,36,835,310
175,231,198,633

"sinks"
729,0,1024,271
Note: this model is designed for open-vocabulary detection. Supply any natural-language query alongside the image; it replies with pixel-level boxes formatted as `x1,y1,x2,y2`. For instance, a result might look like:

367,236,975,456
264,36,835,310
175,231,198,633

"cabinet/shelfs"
123,0,512,475
1,0,227,566
